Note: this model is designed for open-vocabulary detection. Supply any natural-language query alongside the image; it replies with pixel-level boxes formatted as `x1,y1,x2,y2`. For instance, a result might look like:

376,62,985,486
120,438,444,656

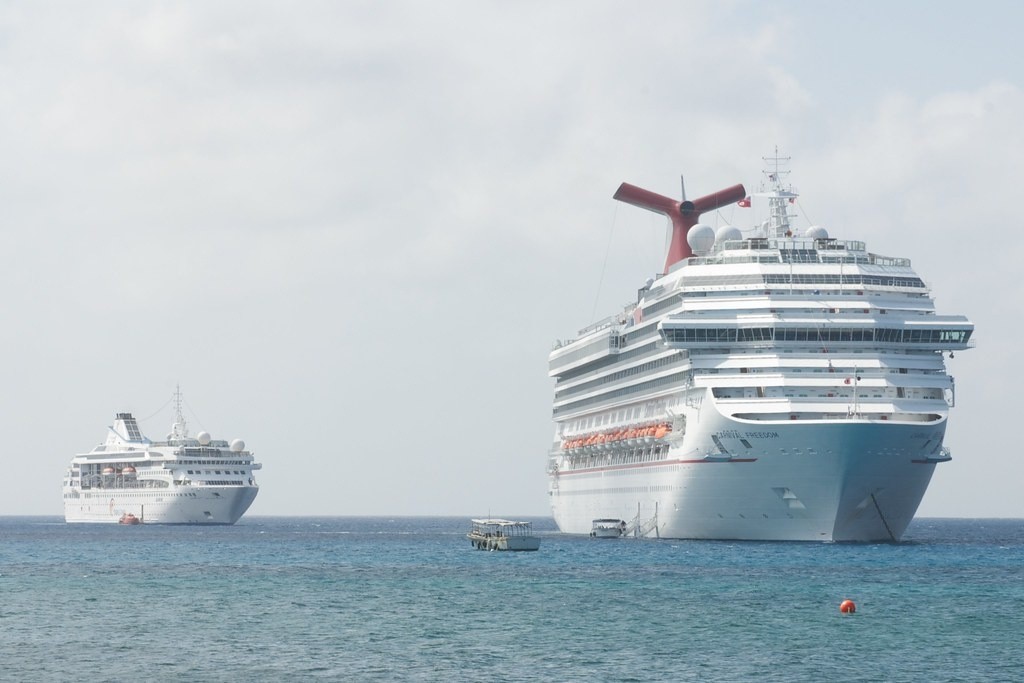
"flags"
789,198,794,204
844,375,851,384
737,196,751,208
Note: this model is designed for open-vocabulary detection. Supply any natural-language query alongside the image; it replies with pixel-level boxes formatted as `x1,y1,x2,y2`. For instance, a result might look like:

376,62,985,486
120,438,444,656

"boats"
122,467,136,476
465,520,542,552
118,513,139,525
562,423,671,458
61,382,263,526
101,467,120,478
591,519,621,538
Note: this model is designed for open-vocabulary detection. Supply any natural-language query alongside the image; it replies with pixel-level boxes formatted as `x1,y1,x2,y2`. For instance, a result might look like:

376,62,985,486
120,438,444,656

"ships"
543,144,974,543
